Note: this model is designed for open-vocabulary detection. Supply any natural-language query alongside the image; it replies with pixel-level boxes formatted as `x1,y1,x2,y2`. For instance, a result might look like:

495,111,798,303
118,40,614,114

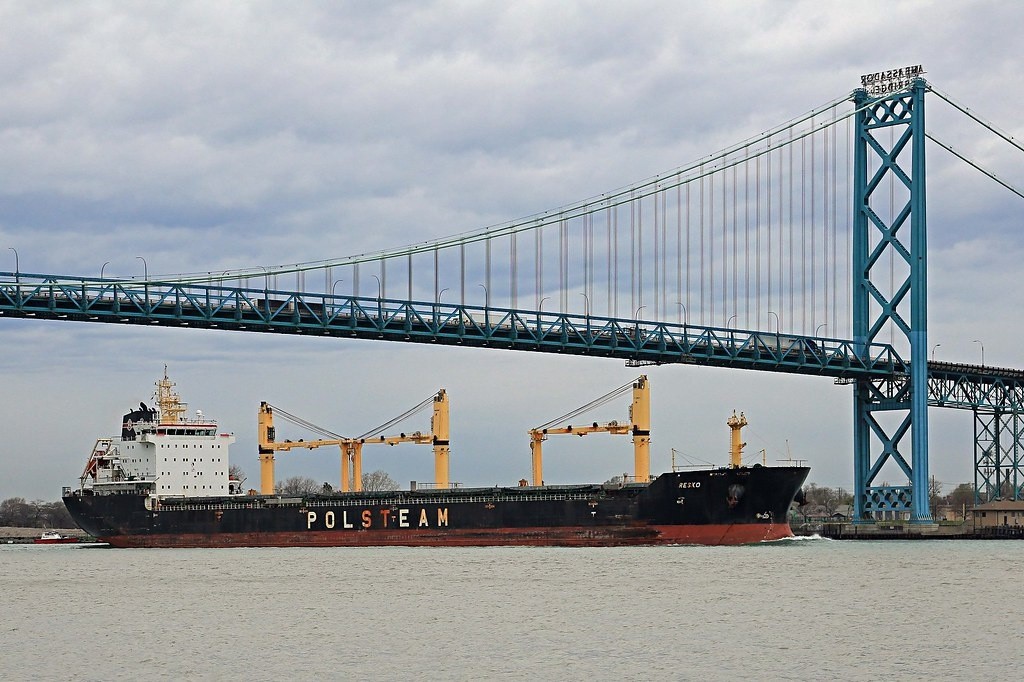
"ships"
61,361,817,549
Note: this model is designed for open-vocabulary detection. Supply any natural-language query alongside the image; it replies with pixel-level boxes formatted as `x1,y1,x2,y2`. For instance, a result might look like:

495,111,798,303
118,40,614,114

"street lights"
636,305,649,322
260,265,268,290
540,296,554,321
332,280,343,295
767,311,781,334
931,343,940,362
438,287,450,323
478,284,490,308
974,339,985,368
579,293,590,318
815,323,827,346
101,261,110,291
8,248,19,283
371,274,382,300
676,302,688,325
727,314,736,339
135,256,147,281
